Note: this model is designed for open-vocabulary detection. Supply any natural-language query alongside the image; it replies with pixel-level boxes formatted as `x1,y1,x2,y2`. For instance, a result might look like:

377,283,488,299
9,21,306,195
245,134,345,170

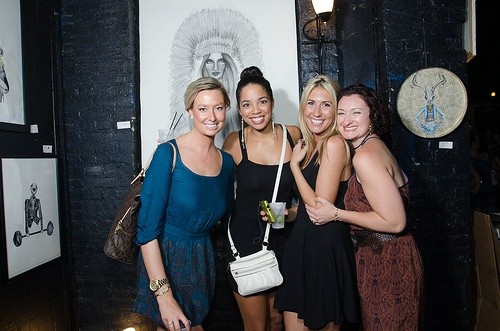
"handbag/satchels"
227,250,284,296
102,141,178,266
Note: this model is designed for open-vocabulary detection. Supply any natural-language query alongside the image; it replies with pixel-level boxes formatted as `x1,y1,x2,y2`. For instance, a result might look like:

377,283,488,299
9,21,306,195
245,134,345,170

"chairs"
469,210,500,331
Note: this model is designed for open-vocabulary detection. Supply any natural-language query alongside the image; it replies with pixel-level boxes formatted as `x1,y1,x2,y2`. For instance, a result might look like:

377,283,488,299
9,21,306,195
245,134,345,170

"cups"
269,203,286,229
158,129,174,144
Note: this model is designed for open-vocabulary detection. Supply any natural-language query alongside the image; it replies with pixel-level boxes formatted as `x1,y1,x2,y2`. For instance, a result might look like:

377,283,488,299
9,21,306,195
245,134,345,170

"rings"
311,220,316,223
168,321,173,324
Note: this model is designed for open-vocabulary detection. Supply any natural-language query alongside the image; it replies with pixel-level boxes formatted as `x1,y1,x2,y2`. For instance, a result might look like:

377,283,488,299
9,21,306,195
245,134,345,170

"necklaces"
353,131,371,150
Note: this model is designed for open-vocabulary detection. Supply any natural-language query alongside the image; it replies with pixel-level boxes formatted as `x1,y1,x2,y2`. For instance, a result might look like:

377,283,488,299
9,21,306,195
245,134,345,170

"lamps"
301,0,336,75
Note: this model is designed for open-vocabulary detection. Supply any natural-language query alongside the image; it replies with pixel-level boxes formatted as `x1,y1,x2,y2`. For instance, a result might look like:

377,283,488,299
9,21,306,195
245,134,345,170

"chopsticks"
166,112,183,138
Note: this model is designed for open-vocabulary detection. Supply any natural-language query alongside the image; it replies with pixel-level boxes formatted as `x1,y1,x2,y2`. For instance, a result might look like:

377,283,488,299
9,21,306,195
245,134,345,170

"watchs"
150,278,169,292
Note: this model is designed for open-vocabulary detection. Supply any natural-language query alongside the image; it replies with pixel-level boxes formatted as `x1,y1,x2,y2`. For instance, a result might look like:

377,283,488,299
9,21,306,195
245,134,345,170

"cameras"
259,200,277,223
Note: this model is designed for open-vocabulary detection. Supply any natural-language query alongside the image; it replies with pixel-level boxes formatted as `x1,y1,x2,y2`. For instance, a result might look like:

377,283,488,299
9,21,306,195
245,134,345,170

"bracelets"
155,284,170,296
335,209,339,221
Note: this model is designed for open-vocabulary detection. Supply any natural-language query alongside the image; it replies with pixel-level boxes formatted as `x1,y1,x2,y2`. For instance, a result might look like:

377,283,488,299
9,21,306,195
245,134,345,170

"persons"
222,67,303,331
260,76,362,331
133,79,234,331
305,85,424,331
